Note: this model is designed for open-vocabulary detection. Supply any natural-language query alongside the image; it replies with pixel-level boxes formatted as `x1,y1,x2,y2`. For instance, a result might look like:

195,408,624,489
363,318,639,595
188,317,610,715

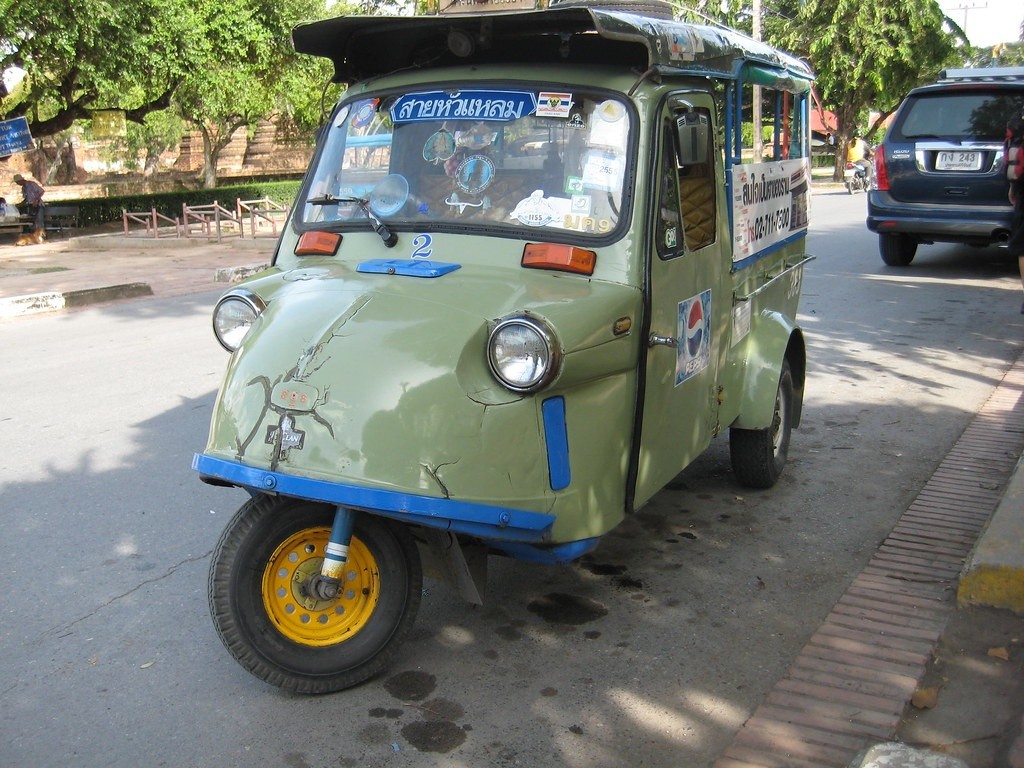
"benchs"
15,205,80,235
404,169,716,252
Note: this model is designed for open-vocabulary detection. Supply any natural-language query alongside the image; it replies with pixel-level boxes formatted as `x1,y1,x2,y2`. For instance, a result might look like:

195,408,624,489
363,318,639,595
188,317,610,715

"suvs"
865,67,1023,275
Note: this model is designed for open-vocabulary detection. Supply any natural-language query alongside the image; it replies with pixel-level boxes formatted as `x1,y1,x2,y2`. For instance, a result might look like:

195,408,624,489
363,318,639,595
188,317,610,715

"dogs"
13,225,47,246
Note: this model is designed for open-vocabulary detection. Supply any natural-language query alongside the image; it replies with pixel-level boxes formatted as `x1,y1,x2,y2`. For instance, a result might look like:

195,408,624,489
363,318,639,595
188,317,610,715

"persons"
826,133,834,146
13,174,45,237
1004,106,1024,312
847,129,876,186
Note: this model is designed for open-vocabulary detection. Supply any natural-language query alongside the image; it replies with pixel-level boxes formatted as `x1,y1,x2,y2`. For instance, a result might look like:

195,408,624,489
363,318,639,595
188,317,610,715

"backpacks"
1004,107,1024,182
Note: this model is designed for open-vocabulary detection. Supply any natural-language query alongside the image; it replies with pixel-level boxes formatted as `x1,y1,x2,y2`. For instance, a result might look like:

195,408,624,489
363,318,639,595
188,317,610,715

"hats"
14,174,25,182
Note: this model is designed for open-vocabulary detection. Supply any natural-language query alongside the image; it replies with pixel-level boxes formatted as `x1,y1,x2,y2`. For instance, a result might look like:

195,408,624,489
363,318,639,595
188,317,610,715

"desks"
0,222,34,244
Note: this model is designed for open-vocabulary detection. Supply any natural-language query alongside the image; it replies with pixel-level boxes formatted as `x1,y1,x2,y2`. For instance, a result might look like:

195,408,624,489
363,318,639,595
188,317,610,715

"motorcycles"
845,145,876,194
186,5,814,682
